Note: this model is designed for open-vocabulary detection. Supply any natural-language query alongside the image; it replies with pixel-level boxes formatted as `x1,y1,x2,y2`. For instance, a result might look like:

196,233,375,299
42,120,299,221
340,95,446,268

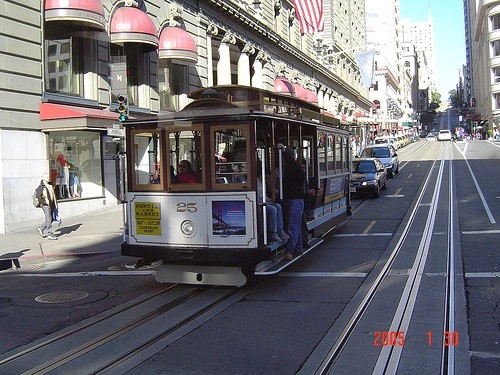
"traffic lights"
116,93,130,123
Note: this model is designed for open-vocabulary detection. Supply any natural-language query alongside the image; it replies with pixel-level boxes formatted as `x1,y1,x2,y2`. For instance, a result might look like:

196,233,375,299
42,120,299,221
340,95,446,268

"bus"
120,85,351,287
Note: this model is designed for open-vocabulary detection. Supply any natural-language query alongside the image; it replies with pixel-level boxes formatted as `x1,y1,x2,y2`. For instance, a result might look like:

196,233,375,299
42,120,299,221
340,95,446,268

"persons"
151,142,317,261
36,173,59,240
49,153,81,198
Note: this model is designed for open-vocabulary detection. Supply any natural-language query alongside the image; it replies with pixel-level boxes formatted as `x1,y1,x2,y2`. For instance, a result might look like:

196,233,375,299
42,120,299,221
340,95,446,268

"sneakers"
36,227,46,238
48,235,58,240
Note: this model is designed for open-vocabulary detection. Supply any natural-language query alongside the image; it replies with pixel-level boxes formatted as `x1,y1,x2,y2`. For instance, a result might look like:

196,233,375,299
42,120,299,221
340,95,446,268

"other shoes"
284,253,292,260
282,234,290,239
305,243,311,248
274,237,281,242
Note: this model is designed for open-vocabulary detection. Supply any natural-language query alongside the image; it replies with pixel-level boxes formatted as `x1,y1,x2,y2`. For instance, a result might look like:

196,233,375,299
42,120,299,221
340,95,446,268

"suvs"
419,131,426,138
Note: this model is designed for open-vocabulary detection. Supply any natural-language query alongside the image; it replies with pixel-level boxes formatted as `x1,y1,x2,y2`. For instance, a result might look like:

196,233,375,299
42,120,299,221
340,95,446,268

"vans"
438,129,451,141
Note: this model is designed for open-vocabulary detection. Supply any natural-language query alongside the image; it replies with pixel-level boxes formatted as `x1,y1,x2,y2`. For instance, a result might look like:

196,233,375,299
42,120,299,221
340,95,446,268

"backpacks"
33,189,45,208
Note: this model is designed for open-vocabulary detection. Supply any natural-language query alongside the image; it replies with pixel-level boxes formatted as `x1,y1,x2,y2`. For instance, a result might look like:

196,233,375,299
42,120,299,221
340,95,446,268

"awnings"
109,5,159,53
275,76,319,104
158,26,198,68
44,0,105,30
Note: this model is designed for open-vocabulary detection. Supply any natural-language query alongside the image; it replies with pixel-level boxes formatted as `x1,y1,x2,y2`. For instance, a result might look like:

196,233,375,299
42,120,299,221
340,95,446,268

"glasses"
179,164,190,168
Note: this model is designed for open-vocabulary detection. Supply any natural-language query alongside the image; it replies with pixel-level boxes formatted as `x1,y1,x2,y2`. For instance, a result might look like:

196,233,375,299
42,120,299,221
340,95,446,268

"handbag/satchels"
52,205,58,221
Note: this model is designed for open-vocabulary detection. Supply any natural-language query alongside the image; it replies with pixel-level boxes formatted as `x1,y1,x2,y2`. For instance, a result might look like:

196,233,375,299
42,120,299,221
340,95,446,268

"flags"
354,49,377,88
291,0,325,34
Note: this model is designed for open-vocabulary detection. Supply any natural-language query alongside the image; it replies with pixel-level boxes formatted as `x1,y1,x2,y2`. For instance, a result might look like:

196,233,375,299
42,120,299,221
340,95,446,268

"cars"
374,133,419,151
426,135,437,142
360,142,399,178
350,157,389,199
